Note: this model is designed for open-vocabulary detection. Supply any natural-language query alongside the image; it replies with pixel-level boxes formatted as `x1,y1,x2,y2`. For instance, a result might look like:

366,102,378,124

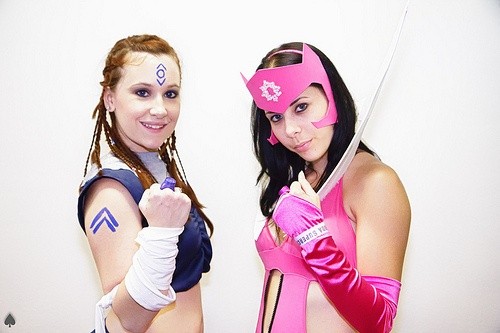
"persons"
78,35,214,333
240,42,411,333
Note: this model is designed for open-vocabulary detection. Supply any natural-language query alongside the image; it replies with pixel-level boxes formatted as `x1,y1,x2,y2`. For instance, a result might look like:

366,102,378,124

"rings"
279,186,290,195
160,176,176,192
